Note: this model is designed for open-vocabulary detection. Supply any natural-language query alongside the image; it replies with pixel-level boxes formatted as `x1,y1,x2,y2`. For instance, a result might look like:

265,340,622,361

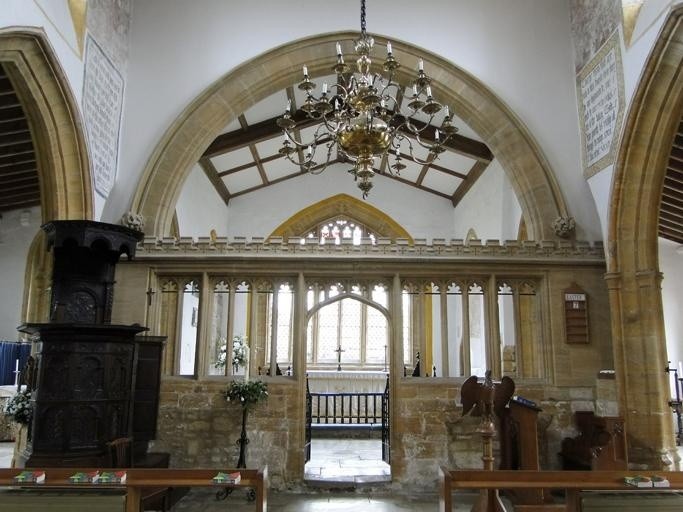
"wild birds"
459,368,516,419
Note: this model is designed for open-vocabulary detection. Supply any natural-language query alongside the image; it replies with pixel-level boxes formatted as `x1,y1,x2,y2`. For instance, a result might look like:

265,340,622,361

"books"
98,470,127,483
71,470,99,483
13,470,46,483
213,472,241,484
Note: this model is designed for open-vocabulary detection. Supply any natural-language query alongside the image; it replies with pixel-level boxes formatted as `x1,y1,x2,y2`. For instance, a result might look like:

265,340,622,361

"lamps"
275,0,460,194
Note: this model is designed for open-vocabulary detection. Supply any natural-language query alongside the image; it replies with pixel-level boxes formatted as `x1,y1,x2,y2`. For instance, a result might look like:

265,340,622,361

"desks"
426,465,683,512
0,463,271,512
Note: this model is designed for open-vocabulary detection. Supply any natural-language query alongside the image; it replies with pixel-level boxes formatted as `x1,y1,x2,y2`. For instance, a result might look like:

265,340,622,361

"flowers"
215,333,253,371
